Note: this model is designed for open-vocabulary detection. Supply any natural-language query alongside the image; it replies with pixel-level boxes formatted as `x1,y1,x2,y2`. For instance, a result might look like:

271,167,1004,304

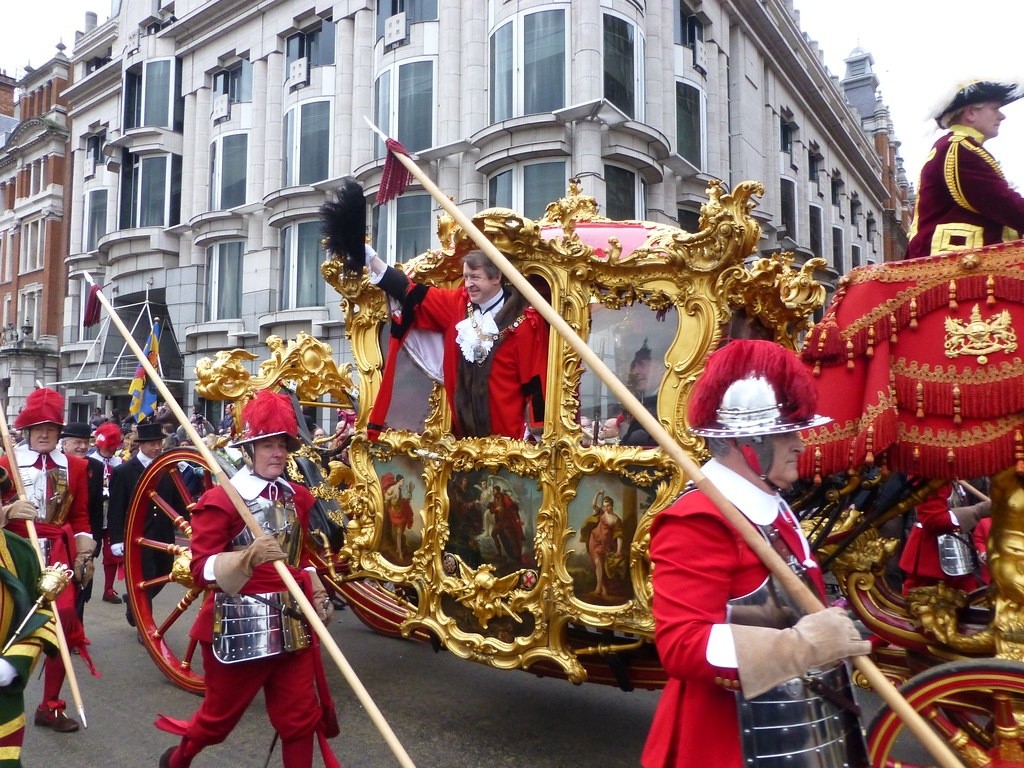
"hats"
59,422,95,439
132,423,168,442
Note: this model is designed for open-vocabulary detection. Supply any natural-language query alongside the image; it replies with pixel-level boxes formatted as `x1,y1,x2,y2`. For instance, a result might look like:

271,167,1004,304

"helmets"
93,423,122,448
14,387,65,427
228,390,302,453
688,339,835,439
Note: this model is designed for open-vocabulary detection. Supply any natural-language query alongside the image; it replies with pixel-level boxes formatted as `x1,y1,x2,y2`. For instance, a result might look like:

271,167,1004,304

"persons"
362,250,550,443
637,336,872,768
897,476,998,607
580,344,666,448
3,393,354,768
903,78,1023,259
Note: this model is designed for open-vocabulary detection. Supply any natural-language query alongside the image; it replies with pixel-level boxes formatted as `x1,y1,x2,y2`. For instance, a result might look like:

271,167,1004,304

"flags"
129,323,163,424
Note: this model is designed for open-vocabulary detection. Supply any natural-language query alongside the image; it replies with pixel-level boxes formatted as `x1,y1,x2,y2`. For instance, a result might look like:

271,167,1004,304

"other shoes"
121,593,136,627
102,592,122,603
34,704,80,732
160,747,179,768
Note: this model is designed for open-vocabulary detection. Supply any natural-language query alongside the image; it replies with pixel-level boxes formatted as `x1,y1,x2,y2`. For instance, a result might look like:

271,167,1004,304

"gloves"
213,536,290,598
730,602,794,629
950,501,993,534
110,542,124,556
0,501,40,528
308,571,335,629
365,243,377,266
729,604,872,701
74,534,97,590
